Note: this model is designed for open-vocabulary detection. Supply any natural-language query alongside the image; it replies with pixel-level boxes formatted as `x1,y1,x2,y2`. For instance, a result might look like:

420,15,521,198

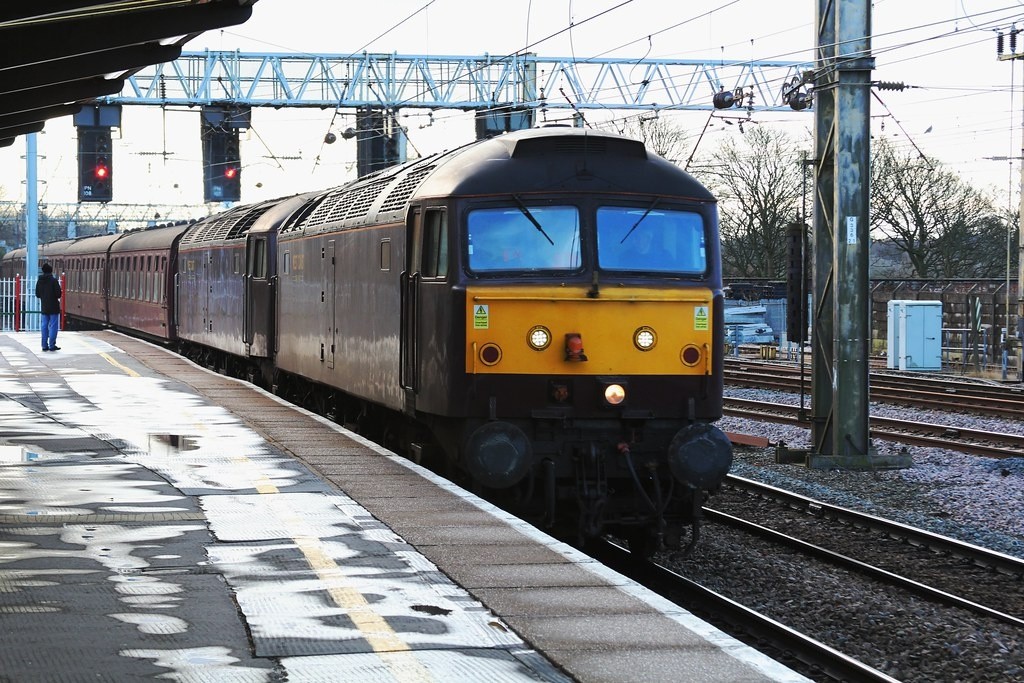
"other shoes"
50,346,61,351
42,347,49,352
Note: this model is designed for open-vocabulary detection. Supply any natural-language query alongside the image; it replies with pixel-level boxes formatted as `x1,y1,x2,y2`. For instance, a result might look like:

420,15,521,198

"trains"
3,125,736,579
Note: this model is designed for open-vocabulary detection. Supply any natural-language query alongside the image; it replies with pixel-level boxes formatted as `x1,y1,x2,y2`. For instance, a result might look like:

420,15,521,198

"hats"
42,263,52,273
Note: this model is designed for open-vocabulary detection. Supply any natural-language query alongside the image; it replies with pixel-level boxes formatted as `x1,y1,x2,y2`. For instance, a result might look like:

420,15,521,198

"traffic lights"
95,134,112,195
222,134,240,190
381,134,399,170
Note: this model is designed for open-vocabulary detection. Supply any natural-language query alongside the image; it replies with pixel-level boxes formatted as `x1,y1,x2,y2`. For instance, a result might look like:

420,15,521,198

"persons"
619,225,666,270
36,262,63,351
